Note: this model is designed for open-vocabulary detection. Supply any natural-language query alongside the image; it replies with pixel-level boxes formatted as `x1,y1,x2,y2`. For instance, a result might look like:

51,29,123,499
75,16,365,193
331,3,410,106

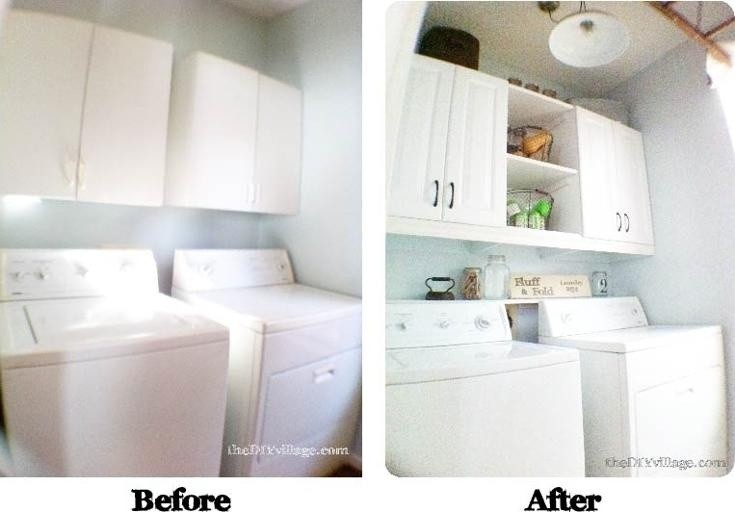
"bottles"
460,267,482,299
481,255,512,300
592,271,609,297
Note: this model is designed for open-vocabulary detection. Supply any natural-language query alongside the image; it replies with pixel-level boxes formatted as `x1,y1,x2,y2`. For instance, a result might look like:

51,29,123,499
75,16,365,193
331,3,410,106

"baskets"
508,126,553,162
508,188,554,230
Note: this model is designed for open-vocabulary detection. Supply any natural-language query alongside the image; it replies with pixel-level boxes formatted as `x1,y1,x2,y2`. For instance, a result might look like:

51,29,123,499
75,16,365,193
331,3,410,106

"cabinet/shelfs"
383,52,656,257
1,8,303,218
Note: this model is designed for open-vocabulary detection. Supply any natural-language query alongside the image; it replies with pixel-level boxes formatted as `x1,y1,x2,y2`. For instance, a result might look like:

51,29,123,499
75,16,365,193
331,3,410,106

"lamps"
537,1,632,74
